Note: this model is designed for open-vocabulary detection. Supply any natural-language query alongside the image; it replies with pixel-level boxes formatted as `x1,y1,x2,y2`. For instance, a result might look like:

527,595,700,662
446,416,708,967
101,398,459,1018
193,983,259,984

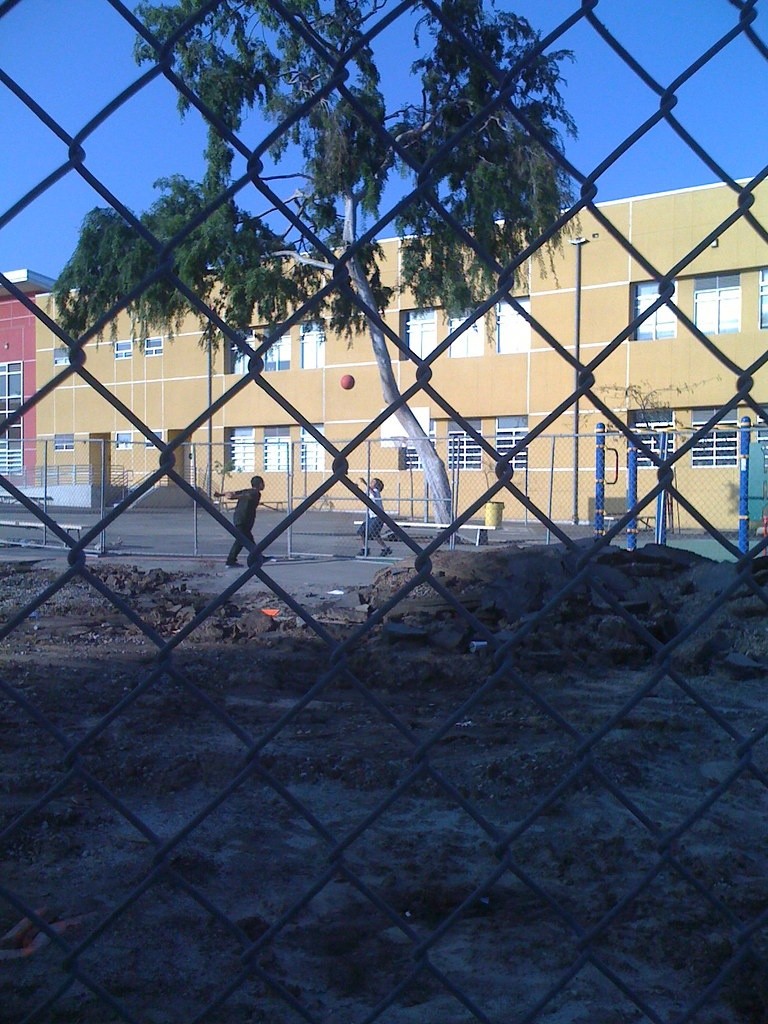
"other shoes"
226,562,244,569
380,547,393,557
358,547,370,556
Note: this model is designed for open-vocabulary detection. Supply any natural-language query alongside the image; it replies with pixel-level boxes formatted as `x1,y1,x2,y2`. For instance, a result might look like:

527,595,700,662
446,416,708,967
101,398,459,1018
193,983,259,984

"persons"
225,475,275,567
357,477,393,557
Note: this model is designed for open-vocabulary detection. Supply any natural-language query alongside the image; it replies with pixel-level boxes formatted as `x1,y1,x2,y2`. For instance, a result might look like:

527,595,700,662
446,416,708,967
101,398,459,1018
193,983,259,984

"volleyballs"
340,374,356,390
